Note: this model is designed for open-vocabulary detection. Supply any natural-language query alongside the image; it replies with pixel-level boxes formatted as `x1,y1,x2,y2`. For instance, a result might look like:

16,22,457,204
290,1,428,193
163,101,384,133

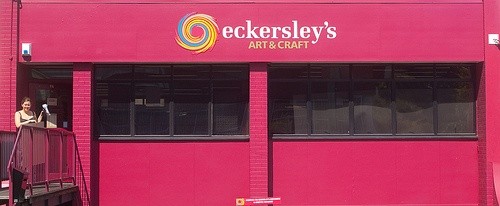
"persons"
16,98,44,143
